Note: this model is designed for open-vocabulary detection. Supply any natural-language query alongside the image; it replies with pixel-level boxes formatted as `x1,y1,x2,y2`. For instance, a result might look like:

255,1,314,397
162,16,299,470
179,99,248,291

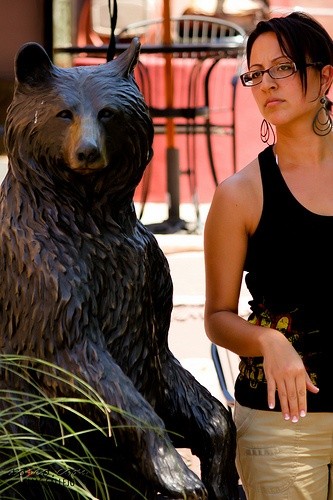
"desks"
54,44,242,233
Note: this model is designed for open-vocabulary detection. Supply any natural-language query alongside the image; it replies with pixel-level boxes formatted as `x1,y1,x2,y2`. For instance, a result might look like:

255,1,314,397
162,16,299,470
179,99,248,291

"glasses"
240,63,313,87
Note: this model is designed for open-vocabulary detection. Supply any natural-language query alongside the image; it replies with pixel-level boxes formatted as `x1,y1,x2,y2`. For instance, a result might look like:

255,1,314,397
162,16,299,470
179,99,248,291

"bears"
0,37,240,500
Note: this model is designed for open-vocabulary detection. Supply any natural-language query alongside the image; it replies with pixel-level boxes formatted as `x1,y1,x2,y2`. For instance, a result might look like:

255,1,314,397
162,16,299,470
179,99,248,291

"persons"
203,11,333,500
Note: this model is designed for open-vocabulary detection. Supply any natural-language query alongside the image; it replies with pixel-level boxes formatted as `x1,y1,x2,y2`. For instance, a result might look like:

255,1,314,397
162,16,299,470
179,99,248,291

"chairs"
114,15,248,235
